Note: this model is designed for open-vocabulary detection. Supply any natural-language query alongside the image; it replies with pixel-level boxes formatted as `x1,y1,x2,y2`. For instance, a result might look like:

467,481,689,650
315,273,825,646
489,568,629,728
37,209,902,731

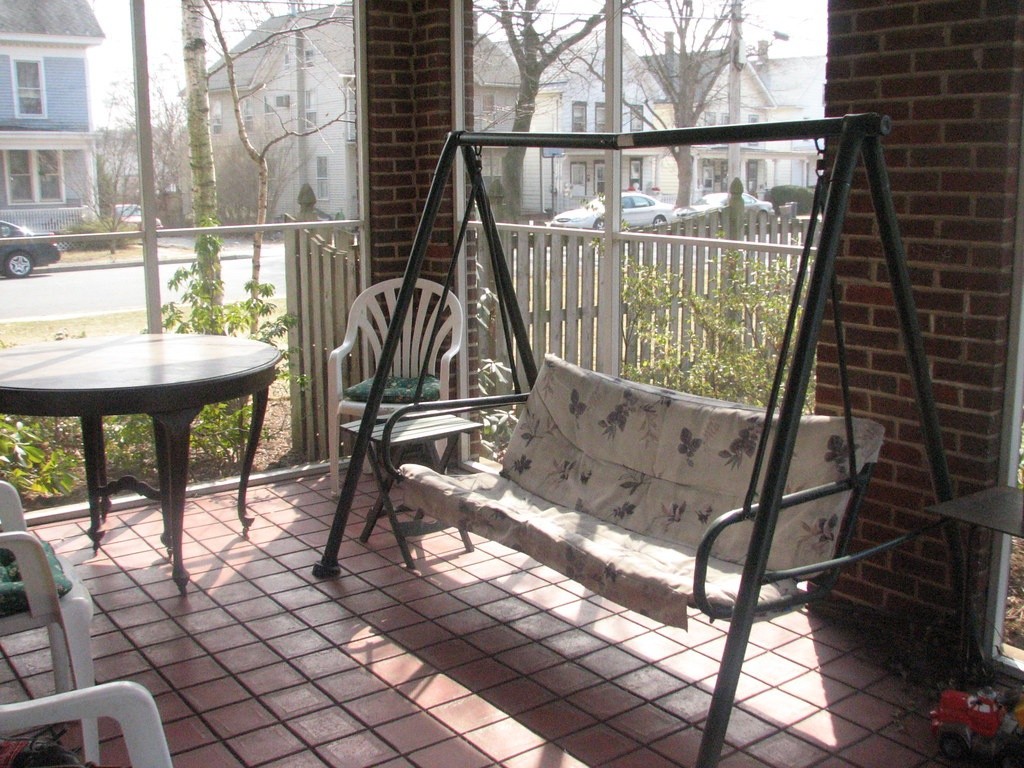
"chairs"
326,277,464,498
0,481,174,768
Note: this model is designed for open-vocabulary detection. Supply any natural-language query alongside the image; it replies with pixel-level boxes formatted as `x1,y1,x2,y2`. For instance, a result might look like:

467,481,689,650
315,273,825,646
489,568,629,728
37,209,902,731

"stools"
338,412,483,570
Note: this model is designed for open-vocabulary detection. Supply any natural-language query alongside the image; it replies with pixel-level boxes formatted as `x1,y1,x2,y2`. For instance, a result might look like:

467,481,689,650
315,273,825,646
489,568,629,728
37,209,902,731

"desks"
0,332,282,596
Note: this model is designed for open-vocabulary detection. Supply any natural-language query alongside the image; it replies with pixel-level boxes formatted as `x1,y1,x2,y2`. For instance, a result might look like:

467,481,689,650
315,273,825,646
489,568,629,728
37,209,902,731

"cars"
115,204,164,234
672,191,775,225
549,190,678,231
0,220,61,280
